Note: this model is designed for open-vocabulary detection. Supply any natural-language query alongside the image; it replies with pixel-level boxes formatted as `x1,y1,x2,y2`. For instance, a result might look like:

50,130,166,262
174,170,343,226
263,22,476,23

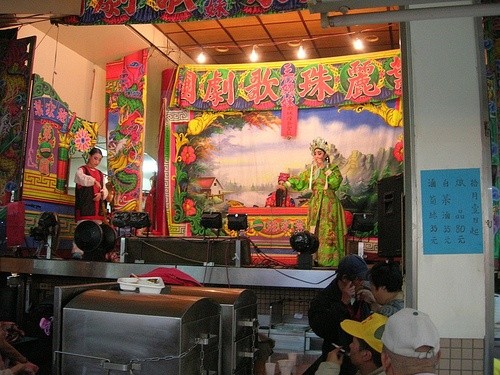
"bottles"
11,191,15,202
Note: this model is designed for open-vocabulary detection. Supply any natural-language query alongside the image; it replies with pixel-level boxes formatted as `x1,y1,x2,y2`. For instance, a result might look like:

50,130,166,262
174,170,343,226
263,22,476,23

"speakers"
123,237,251,268
377,173,403,258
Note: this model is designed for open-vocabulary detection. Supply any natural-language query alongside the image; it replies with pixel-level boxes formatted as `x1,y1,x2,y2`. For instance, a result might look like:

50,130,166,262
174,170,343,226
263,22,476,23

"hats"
382,307,440,358
308,137,332,190
335,255,369,281
339,313,388,352
278,172,290,181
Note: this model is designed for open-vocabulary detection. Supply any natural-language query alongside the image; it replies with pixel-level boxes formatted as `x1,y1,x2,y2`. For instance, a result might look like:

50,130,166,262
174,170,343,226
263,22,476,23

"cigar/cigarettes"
331,341,345,354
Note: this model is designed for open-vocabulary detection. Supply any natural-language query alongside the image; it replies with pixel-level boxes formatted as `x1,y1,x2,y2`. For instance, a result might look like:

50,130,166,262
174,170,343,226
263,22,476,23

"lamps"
200,212,222,229
226,213,248,230
113,211,152,229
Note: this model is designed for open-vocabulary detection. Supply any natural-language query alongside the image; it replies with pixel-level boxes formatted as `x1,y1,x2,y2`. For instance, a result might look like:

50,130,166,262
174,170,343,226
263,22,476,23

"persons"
0,331,41,375
71,147,110,260
283,135,347,268
314,311,388,374
264,171,296,209
364,261,404,316
380,307,442,375
302,253,370,375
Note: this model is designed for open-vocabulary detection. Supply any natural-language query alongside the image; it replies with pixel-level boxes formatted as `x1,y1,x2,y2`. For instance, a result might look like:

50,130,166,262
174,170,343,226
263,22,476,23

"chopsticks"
131,273,141,281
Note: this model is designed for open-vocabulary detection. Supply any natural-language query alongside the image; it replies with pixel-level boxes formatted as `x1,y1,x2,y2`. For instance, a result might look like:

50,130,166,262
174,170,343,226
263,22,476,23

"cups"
265,362,276,375
277,360,293,375
288,353,297,366
